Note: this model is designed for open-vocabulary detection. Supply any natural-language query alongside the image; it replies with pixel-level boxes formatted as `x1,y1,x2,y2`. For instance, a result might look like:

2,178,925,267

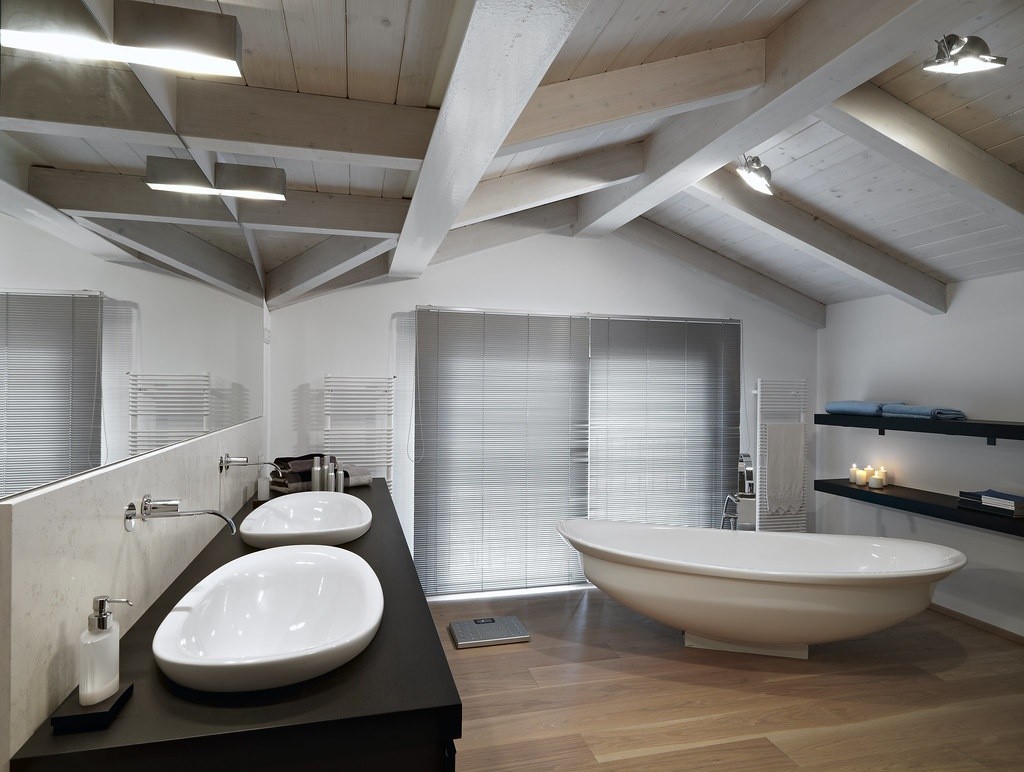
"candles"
869,471,883,489
849,464,858,483
856,469,866,485
875,466,887,485
864,465,875,482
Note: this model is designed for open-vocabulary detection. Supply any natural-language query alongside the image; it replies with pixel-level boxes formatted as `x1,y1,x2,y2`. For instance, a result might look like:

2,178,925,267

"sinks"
151,544,384,693
239,490,373,549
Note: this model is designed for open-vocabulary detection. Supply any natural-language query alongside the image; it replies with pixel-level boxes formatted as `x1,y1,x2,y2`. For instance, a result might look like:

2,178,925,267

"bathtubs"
555,515,968,660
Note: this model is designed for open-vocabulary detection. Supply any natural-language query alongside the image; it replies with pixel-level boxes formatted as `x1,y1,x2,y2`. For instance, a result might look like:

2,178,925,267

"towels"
267,453,351,499
824,400,969,422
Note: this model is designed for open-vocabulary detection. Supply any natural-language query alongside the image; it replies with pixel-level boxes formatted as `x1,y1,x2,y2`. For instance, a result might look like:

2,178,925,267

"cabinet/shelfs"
814,478,1024,537
813,399,1024,447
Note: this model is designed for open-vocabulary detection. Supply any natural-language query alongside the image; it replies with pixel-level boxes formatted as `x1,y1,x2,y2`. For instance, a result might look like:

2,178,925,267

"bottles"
327,462,335,492
337,461,345,494
312,455,322,490
321,456,333,491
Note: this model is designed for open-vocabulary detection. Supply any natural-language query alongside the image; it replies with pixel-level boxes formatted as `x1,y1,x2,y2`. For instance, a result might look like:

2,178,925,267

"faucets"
219,454,283,478
718,492,740,530
123,492,238,536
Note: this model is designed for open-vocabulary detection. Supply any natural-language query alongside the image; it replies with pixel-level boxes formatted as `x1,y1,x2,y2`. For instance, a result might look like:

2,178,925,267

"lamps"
146,155,286,203
0,0,244,78
922,34,1008,75
735,154,774,197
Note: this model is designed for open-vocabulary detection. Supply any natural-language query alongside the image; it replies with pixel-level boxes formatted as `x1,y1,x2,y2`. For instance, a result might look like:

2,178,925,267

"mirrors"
1,177,265,503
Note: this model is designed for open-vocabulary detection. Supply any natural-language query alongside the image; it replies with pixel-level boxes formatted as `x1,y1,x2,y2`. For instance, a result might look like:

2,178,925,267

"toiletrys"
311,455,345,493
78,595,135,707
257,462,271,501
